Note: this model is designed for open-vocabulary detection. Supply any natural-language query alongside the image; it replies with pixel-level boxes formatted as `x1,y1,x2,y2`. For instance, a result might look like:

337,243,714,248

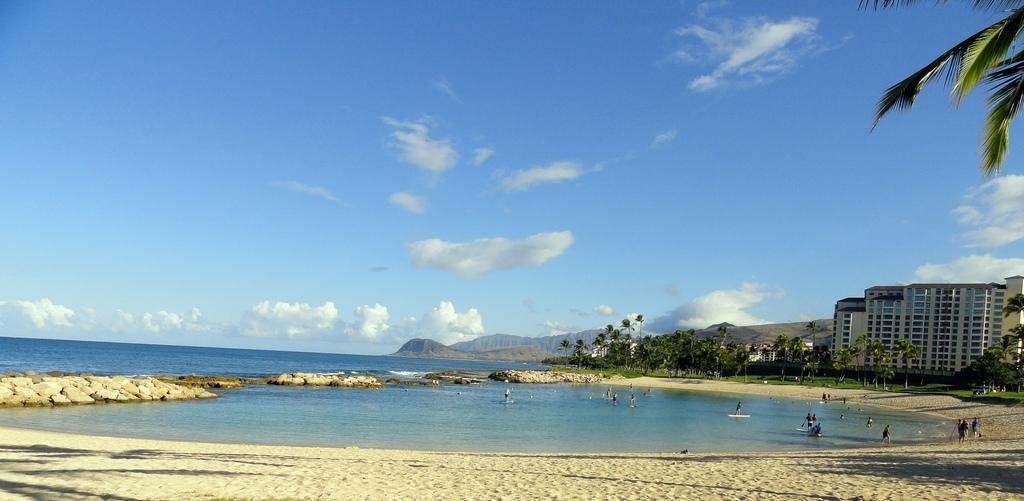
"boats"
728,414,821,437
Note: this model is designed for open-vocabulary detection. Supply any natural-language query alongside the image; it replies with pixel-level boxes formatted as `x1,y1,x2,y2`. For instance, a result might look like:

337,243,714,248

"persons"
822,393,831,400
958,419,969,442
881,424,891,444
795,376,800,382
866,417,873,428
736,402,741,415
806,413,821,436
605,382,635,407
972,417,980,438
505,387,510,399
